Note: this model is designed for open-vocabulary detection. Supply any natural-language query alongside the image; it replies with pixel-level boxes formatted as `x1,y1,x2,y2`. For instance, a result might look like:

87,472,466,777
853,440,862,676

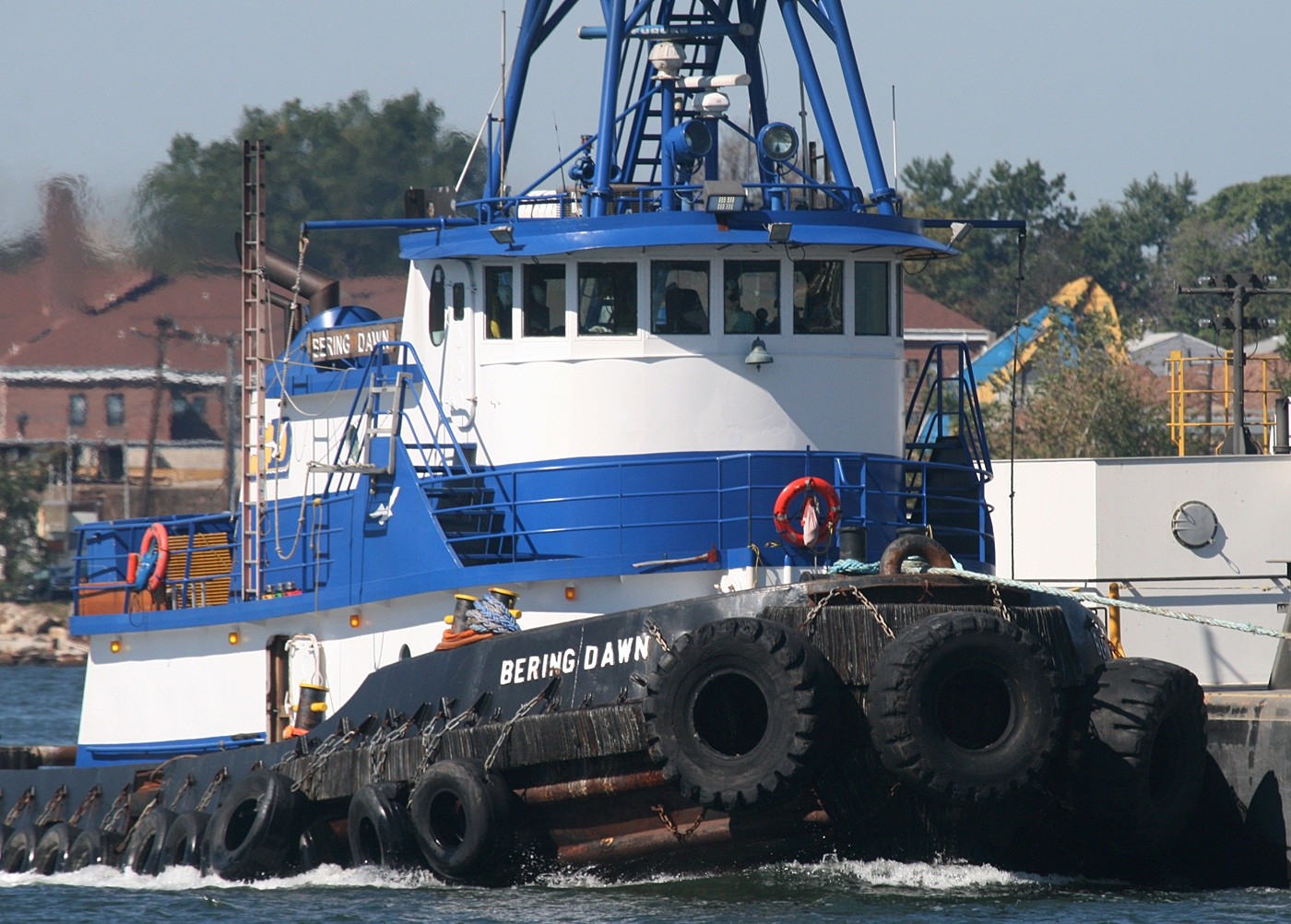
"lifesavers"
1,827,34,874
65,829,116,870
1085,657,1208,834
641,617,834,808
872,615,1059,800
347,785,404,870
281,824,337,876
775,477,840,546
409,761,499,880
34,823,72,877
200,805,223,876
140,523,169,590
121,809,167,875
158,813,200,873
210,768,292,880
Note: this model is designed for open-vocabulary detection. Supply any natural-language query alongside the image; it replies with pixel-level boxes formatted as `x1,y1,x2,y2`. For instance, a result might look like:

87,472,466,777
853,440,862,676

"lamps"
744,346,772,364
702,181,747,214
758,122,799,176
948,222,973,247
666,121,713,176
761,221,793,243
490,225,515,252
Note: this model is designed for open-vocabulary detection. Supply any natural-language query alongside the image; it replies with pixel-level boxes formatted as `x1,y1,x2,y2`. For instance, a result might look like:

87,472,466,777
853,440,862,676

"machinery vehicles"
912,275,1130,456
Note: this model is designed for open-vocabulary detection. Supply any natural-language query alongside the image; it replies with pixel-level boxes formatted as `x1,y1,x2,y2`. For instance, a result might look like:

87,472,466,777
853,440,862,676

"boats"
0,2,1213,889
